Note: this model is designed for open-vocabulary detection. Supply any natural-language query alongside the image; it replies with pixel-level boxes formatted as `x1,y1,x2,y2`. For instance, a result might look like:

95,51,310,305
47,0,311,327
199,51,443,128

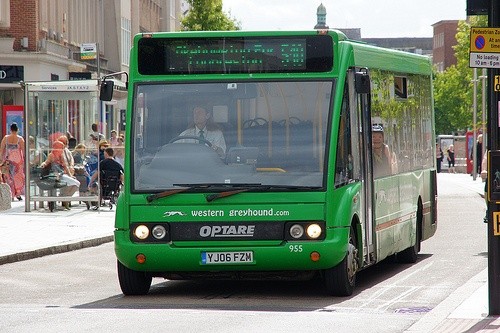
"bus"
99,29,438,297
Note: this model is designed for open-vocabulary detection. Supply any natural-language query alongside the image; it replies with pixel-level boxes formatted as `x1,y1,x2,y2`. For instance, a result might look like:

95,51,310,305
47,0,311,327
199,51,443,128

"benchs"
34,176,67,212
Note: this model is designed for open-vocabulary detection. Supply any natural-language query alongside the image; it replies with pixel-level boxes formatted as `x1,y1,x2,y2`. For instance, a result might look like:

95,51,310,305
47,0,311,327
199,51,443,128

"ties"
198,131,205,145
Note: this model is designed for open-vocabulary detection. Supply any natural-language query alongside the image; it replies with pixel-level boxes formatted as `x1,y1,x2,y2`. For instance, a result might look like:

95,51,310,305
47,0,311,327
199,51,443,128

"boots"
452,167,457,174
448,167,451,174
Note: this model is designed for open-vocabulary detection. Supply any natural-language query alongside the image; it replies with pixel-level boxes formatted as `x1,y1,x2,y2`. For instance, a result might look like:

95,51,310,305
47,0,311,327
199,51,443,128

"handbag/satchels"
440,150,444,159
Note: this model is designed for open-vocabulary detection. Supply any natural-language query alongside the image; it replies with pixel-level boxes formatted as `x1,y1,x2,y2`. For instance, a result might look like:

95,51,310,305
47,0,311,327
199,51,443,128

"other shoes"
17,196,22,201
64,204,71,210
56,207,67,211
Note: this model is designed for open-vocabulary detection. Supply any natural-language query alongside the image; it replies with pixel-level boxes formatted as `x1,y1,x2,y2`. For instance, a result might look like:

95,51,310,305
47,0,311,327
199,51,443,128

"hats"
99,139,112,146
372,124,384,132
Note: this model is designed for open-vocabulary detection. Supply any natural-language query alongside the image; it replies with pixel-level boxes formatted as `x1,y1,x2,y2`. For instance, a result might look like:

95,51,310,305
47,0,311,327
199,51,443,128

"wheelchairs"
86,165,124,211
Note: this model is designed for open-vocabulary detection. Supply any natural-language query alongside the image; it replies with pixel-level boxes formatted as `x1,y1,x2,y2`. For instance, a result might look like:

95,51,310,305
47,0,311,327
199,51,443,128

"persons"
0,122,125,211
436,133,489,224
370,124,397,179
174,103,227,160
